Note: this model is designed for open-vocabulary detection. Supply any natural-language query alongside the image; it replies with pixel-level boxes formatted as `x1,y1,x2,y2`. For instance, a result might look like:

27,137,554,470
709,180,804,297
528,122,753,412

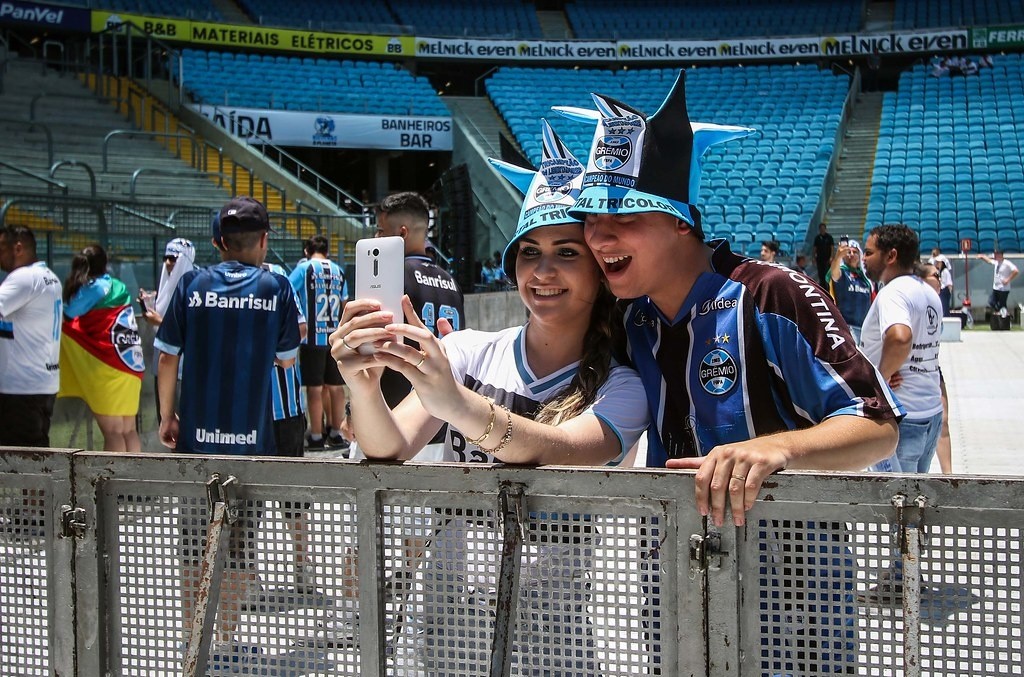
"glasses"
163,255,177,262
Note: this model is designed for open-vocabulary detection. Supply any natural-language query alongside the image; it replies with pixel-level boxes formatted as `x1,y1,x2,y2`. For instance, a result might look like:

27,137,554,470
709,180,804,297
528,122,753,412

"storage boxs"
940,317,961,341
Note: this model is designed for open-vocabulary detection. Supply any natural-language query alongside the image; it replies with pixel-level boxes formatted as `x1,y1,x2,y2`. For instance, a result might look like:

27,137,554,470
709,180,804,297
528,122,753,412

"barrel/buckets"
990,315,1011,331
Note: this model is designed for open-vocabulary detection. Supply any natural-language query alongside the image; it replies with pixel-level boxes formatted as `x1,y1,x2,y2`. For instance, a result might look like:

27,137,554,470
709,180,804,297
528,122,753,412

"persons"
812,223,834,280
914,262,952,474
62,244,146,453
928,52,992,77
830,239,877,345
978,249,1019,308
480,252,506,285
136,237,196,427
551,69,907,677
0,223,63,447
859,225,943,605
929,247,953,317
327,192,465,634
153,196,300,669
287,234,345,449
211,214,324,599
328,118,652,677
761,242,778,263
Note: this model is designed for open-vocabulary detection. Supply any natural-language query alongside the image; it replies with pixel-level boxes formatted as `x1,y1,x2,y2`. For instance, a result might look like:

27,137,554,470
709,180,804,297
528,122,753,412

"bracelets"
466,396,512,455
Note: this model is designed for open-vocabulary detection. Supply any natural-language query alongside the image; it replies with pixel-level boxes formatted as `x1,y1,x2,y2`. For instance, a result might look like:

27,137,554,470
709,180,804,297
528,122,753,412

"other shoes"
211,642,263,670
291,613,360,647
303,435,324,450
857,584,927,605
239,576,265,610
327,434,344,446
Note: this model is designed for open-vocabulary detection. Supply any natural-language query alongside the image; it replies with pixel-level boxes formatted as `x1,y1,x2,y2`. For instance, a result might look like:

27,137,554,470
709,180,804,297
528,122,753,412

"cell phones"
355,236,404,354
840,236,848,248
139,298,148,313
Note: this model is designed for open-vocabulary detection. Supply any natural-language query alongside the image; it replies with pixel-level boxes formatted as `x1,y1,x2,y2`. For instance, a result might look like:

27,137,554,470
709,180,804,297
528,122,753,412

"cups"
142,291,155,310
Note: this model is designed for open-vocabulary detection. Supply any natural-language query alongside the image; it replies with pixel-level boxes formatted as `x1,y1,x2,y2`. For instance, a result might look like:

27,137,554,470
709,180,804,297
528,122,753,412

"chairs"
103,0,1023,258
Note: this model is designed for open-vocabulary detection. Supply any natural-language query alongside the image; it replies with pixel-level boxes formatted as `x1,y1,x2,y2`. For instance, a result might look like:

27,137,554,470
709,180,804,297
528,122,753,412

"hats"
219,197,279,235
848,240,861,250
552,68,756,238
167,238,195,263
487,116,591,286
935,253,952,270
213,213,221,240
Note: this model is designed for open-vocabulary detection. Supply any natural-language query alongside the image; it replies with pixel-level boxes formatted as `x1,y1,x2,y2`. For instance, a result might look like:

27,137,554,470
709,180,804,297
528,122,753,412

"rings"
417,354,425,367
731,475,745,481
343,340,352,350
